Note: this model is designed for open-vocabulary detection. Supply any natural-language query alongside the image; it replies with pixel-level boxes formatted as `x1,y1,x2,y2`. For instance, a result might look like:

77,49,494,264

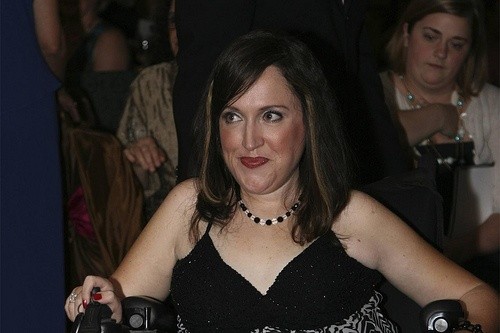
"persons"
66,25,500,333
357,0,499,293
0,1,69,332
63,0,413,183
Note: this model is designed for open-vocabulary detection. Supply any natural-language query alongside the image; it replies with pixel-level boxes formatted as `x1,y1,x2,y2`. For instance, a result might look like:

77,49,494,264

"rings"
68,293,76,303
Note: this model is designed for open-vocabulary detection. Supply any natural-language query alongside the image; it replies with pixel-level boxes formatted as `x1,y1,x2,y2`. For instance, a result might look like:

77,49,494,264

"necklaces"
232,189,306,226
399,73,465,143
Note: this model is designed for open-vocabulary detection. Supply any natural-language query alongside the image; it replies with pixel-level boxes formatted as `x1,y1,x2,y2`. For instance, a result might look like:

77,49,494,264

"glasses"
165,12,176,29
